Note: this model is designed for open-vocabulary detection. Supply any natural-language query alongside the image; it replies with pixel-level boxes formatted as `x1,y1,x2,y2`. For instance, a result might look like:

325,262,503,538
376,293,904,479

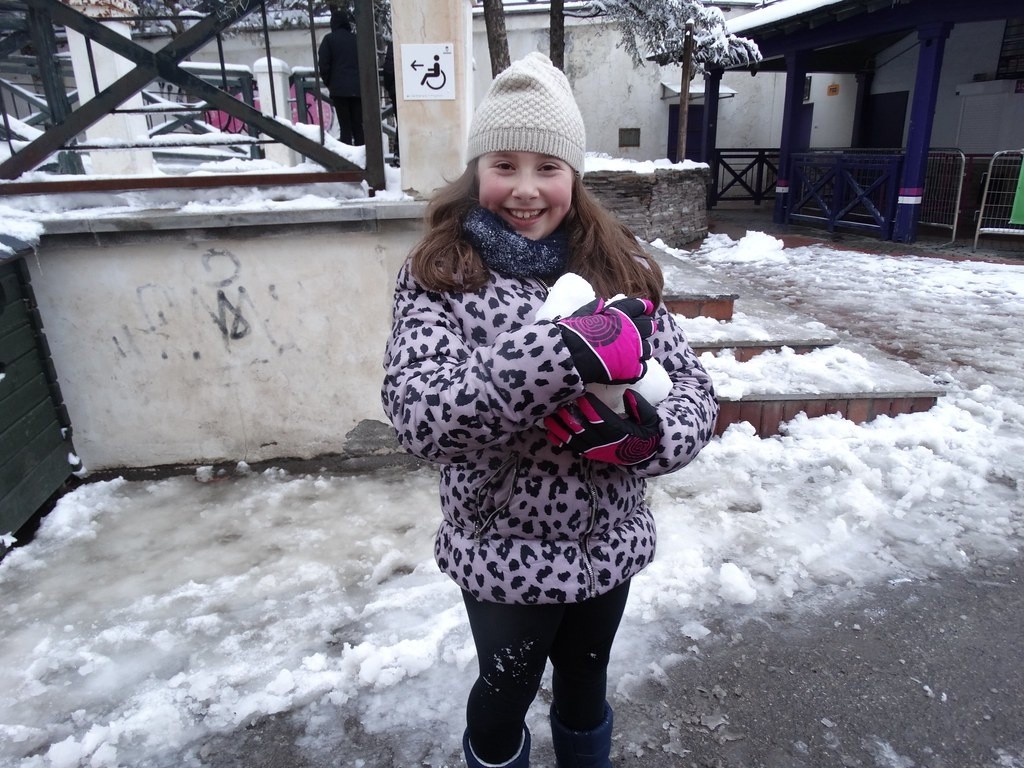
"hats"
465,51,587,179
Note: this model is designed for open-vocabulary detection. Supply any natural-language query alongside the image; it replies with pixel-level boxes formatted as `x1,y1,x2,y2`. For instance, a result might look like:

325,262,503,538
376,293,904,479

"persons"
318,12,366,146
380,51,717,767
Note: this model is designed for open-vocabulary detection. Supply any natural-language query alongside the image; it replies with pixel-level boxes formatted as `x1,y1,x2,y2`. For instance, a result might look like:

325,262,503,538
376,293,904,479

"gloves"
556,296,657,385
543,388,662,467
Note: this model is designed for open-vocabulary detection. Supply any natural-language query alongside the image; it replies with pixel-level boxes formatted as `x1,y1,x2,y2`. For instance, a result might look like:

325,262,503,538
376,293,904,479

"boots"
462,722,531,768
549,700,615,768
